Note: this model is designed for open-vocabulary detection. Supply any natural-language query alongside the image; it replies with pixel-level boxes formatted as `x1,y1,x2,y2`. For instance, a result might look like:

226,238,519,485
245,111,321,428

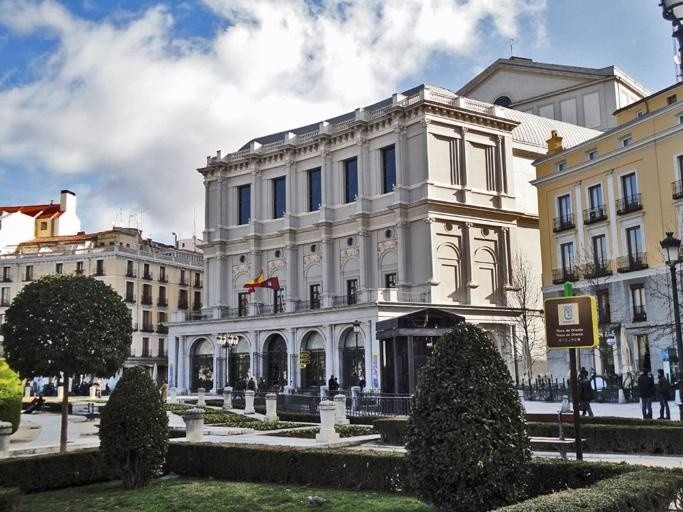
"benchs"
524,435,586,457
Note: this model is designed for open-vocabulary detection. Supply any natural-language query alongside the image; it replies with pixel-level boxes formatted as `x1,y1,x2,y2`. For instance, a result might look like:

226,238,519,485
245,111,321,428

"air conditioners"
588,207,604,221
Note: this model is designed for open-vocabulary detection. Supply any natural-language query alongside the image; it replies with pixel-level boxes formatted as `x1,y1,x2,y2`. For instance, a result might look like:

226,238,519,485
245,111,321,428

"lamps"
604,322,621,346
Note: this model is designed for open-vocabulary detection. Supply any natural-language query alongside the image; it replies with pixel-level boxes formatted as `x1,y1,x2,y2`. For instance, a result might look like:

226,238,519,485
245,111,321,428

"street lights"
658,0,682,74
352,319,362,389
215,333,241,389
659,230,681,422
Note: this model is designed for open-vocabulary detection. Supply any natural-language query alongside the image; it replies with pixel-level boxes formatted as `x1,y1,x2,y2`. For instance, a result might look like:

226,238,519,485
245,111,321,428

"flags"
243,269,279,294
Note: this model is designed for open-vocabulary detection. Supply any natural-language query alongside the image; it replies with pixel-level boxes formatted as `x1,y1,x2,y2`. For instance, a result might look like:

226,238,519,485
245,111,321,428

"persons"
195,376,288,400
22,395,43,413
159,380,168,402
521,366,671,420
43,381,109,397
319,375,339,390
